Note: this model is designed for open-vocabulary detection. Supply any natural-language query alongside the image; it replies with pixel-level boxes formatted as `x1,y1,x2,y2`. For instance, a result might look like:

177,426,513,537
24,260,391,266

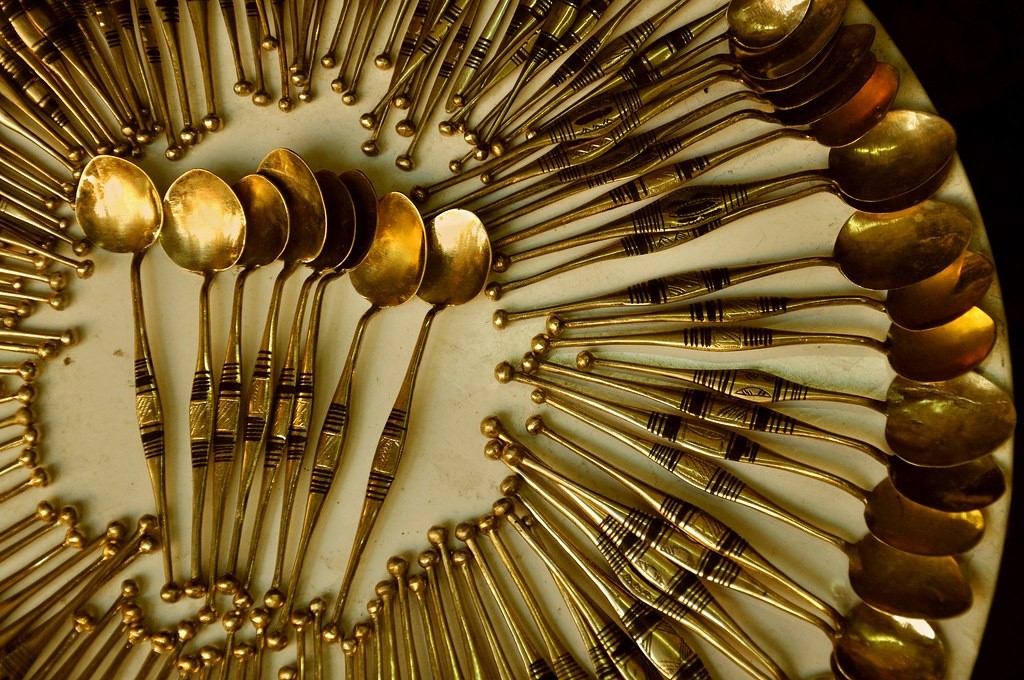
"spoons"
75,0,1014,680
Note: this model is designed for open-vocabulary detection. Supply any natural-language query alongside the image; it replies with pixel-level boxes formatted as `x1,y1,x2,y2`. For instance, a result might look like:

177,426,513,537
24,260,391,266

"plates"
0,0,1013,680
